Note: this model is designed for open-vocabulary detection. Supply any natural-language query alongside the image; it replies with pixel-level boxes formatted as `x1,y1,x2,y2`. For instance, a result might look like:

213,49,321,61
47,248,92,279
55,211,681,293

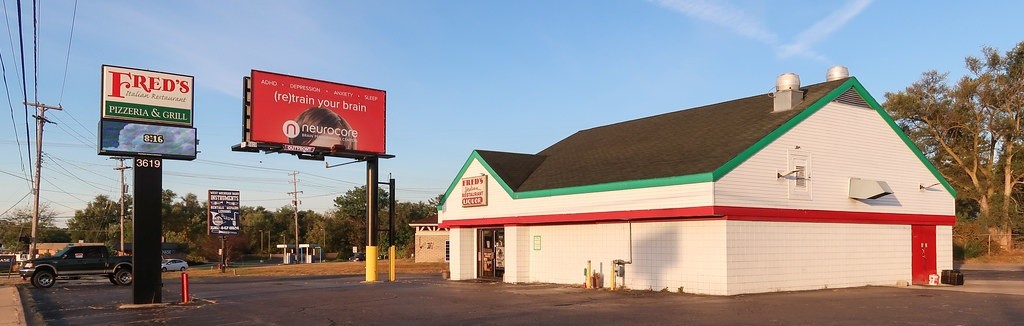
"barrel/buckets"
929,274,939,285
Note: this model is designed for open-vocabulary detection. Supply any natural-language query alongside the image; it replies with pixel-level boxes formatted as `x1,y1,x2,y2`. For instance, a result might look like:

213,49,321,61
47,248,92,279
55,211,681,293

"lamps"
332,144,346,154
778,169,799,177
920,183,939,189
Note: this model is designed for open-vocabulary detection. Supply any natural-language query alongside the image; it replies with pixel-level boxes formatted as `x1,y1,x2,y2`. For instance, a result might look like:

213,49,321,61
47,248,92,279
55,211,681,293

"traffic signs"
209,190,240,235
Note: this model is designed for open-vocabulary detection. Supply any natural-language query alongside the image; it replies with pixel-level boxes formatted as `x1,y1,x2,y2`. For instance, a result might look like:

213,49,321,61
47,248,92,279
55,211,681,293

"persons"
288,107,357,149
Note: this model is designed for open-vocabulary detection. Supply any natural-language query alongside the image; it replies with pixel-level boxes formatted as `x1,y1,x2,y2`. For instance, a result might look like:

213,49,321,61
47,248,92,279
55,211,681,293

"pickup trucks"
19,246,132,289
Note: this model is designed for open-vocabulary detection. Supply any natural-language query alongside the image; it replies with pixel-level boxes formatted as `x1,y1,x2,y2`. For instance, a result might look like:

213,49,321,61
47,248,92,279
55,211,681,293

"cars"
161,259,188,272
349,253,366,262
